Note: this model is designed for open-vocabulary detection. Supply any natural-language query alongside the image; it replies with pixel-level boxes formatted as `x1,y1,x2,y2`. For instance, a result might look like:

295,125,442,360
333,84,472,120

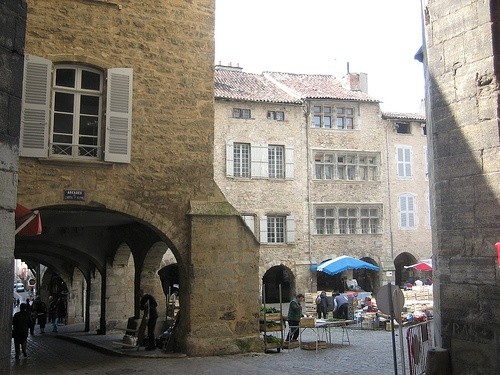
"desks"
288,319,354,354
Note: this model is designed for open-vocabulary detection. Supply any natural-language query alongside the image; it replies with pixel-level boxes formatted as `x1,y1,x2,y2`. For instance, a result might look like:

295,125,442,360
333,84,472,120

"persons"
339,276,347,294
13,297,45,334
315,290,329,319
332,292,349,328
404,276,433,290
285,293,304,342
11,303,33,359
37,303,49,334
140,294,157,351
57,298,67,325
48,296,59,333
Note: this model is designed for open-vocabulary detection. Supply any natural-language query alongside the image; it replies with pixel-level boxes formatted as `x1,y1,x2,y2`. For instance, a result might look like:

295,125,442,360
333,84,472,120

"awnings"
310,254,381,277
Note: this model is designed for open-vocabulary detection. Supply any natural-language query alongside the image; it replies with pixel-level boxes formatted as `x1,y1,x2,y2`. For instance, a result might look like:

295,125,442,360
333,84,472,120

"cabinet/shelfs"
261,284,284,352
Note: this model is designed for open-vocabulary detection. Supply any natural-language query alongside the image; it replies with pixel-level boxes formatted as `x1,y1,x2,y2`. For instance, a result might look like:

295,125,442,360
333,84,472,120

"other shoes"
23,351,27,357
145,345,156,351
15,354,19,358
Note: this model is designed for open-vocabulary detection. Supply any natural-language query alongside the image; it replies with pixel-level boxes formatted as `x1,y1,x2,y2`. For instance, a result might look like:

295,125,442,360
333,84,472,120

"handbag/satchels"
316,294,321,304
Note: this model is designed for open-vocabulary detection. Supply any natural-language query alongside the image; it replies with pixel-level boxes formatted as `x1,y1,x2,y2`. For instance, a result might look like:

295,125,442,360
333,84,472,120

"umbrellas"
403,259,432,285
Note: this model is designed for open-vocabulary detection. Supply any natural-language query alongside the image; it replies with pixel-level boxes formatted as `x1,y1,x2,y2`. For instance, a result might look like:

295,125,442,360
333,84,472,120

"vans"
16,283,24,292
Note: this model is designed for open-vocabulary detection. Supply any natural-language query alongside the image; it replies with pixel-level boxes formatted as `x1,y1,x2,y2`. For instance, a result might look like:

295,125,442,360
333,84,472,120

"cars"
14,284,16,288
